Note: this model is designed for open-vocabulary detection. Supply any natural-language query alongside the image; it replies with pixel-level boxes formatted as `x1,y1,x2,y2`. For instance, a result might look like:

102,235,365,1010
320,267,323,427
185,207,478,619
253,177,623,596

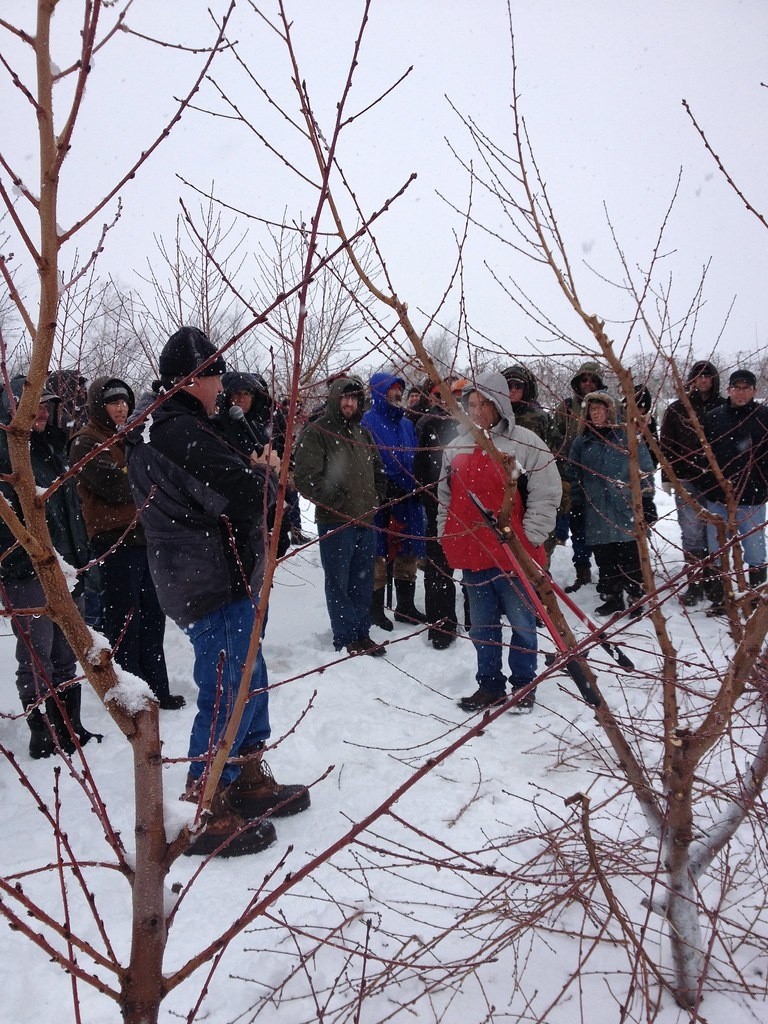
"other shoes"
461,690,507,710
346,638,387,655
159,696,184,710
510,689,535,713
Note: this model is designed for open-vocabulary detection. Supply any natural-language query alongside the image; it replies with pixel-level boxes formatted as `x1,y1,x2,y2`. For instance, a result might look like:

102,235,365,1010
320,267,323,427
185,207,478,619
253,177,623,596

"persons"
295,363,648,655
660,360,727,602
437,374,559,715
561,389,658,621
117,326,310,857
0,369,311,755
694,369,768,615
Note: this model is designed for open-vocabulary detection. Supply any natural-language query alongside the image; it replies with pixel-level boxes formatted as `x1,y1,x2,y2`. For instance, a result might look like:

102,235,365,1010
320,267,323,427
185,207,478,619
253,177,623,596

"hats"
159,326,226,376
39,388,62,404
506,377,525,384
102,380,129,402
730,369,756,386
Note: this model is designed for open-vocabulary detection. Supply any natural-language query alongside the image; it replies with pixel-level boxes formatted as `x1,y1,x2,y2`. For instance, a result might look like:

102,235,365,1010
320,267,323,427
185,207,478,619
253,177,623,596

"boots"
595,591,625,616
22,697,76,759
565,565,591,593
229,740,310,818
371,575,472,650
678,550,704,603
629,593,642,618
179,772,276,857
704,566,729,617
52,684,103,753
747,564,766,608
291,529,311,545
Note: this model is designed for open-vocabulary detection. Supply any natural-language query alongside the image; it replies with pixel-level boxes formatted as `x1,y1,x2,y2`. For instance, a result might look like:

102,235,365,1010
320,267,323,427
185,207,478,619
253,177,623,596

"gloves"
643,497,657,523
570,505,585,528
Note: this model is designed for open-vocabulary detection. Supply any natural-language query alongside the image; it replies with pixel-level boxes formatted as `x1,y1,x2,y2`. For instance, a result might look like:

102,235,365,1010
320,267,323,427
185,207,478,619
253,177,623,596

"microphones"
229,406,264,455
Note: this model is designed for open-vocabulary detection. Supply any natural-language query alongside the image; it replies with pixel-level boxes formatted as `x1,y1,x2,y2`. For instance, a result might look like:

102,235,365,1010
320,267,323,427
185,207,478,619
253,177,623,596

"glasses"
107,401,132,407
508,383,521,389
580,376,597,383
731,384,753,392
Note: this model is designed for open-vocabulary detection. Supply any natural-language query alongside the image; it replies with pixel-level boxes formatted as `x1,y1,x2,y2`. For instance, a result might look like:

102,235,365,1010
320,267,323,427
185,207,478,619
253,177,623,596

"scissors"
468,489,634,707
